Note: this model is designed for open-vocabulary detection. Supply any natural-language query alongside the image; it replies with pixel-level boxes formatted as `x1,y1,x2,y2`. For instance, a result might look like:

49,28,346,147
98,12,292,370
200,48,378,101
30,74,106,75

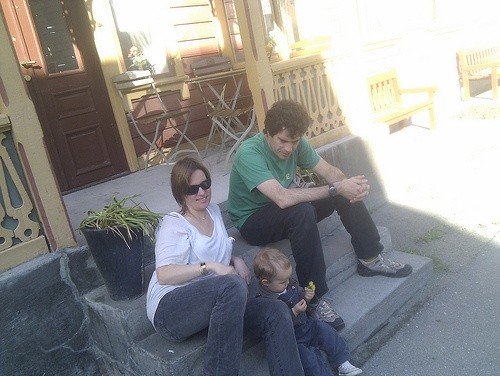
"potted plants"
74,193,164,302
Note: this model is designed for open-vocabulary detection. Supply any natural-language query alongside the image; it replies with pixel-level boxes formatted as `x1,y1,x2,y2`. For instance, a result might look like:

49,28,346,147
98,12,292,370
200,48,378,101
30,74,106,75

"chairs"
111,70,202,171
191,44,273,175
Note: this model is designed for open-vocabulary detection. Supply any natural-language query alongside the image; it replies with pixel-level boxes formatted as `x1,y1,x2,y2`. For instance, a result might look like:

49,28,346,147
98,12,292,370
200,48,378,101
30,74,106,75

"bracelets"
200,262,207,276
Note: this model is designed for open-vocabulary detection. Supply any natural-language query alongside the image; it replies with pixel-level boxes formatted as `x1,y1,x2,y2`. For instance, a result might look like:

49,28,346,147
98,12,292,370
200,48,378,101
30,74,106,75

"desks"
183,68,248,139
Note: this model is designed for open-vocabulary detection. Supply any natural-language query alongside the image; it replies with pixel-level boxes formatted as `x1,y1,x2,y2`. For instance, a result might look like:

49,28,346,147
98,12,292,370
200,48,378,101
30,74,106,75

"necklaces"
199,216,209,220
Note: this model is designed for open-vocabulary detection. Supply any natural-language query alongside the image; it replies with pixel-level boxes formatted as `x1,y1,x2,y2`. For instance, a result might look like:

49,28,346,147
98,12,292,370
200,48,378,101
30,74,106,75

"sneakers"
310,295,345,329
356,251,413,278
337,360,362,376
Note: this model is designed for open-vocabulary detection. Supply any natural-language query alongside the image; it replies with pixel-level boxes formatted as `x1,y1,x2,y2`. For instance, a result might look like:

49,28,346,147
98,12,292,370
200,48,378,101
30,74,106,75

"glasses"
185,179,211,195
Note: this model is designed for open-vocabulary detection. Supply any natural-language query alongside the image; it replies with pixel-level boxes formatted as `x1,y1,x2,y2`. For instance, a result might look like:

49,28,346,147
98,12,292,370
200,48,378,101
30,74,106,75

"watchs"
329,183,336,198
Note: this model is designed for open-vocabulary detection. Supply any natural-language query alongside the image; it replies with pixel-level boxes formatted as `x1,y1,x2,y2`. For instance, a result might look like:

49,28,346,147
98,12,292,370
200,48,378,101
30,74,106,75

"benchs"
457,44,500,102
367,70,438,131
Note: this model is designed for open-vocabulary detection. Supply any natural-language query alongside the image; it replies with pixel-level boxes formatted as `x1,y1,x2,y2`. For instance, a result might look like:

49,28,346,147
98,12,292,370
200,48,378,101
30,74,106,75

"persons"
227,100,413,330
146,157,305,376
254,248,362,376
